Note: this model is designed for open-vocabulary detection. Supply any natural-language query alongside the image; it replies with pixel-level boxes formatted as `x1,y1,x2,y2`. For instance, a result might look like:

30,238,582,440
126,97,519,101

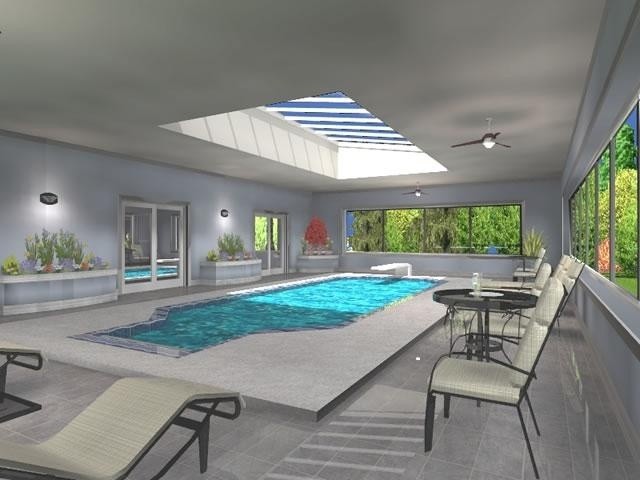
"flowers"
3,228,111,276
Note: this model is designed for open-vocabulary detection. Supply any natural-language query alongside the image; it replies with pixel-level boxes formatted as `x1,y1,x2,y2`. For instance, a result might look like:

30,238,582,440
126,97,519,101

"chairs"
425,244,587,479
1,340,247,480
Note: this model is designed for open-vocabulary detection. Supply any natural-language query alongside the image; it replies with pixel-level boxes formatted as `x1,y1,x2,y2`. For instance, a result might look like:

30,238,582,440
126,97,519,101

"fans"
402,182,430,197
450,116,513,149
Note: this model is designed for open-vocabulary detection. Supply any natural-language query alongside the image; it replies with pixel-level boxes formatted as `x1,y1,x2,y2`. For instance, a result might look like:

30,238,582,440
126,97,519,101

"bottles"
472,272,481,297
